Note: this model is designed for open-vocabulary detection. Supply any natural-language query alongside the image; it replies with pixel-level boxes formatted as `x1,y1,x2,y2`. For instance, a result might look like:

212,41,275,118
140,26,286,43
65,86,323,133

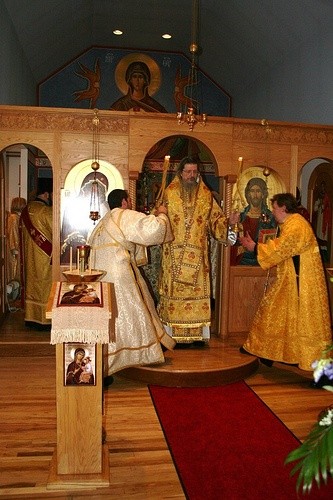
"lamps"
90,114,103,225
262,121,272,220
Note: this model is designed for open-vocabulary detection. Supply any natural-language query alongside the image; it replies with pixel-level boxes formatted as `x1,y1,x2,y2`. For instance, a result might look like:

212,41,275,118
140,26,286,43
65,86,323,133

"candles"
70,247,73,272
77,249,79,273
89,249,92,274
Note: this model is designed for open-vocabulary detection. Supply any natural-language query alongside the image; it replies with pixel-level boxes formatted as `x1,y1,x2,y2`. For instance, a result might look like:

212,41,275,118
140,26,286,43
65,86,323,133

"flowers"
286,345,333,492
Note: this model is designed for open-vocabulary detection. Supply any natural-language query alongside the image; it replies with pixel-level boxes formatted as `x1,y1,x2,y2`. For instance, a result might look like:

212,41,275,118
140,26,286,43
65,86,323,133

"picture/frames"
259,229,277,243
63,342,97,387
57,280,103,307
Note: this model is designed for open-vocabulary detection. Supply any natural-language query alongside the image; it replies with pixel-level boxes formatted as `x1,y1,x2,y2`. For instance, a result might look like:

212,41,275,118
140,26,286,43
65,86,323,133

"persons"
6,184,53,330
236,192,331,371
150,156,240,349
86,188,176,385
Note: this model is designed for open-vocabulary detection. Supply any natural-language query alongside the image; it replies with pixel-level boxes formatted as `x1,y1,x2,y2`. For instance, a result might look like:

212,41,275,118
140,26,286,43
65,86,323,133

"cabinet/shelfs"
228,266,333,336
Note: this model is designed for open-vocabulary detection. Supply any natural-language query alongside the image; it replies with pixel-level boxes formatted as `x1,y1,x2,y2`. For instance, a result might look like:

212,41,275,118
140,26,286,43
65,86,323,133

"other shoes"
310,374,333,388
25,321,52,332
240,346,273,368
108,375,113,385
193,341,205,348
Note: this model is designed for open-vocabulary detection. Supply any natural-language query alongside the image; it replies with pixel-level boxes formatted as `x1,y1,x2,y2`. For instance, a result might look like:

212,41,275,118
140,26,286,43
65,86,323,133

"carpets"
147,378,333,500
